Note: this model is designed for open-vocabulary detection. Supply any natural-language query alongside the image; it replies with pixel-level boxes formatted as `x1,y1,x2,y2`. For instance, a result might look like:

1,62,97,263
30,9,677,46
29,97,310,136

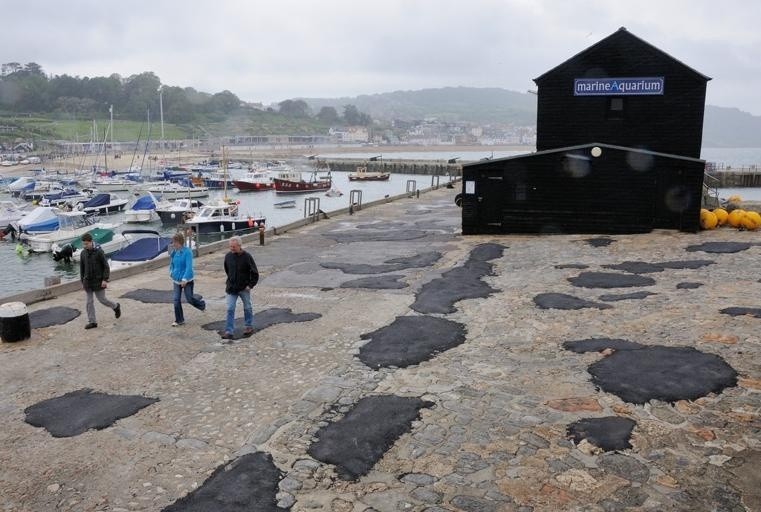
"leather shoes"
242,327,255,336
221,332,234,339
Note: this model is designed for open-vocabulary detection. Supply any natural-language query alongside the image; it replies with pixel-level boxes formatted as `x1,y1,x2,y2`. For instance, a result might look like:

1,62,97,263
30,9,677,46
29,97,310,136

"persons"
222,236,259,339
80,233,121,329
168,233,207,326
50,240,58,258
11,228,33,257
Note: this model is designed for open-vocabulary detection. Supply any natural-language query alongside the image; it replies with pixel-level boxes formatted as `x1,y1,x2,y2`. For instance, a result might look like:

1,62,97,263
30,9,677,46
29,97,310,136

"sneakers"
85,321,98,329
171,320,186,327
114,302,121,318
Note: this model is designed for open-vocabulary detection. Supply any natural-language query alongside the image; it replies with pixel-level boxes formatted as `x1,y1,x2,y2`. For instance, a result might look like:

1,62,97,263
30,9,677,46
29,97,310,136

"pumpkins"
700,207,760,231
